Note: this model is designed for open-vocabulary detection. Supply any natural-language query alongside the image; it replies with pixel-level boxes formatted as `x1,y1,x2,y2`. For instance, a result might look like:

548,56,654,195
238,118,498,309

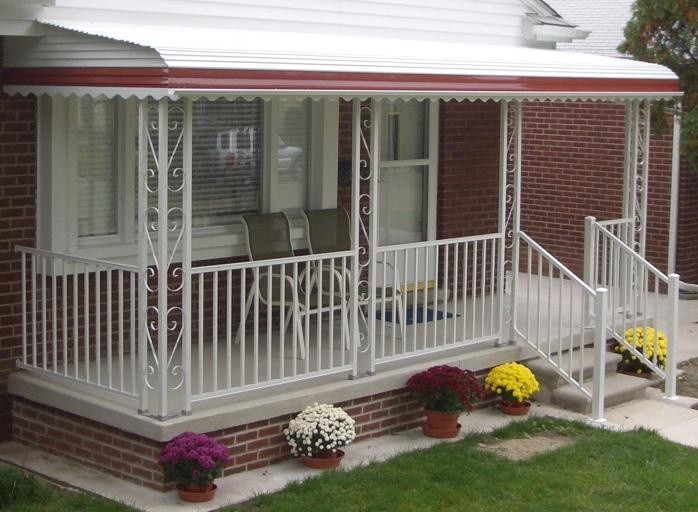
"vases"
177,480,218,503
303,449,346,469
624,353,653,378
499,389,531,415
422,410,461,439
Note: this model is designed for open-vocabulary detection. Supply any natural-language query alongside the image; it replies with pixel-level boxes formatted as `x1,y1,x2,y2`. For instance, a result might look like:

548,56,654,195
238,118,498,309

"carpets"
362,305,461,325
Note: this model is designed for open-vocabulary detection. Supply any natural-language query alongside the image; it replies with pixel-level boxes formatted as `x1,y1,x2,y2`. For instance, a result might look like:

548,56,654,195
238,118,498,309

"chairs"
234,210,350,361
298,206,406,350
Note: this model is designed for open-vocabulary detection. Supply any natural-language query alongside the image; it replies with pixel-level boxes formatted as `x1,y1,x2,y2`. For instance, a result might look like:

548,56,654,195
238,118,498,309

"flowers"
614,324,669,376
484,361,542,404
158,429,231,487
408,365,486,414
282,402,356,458
